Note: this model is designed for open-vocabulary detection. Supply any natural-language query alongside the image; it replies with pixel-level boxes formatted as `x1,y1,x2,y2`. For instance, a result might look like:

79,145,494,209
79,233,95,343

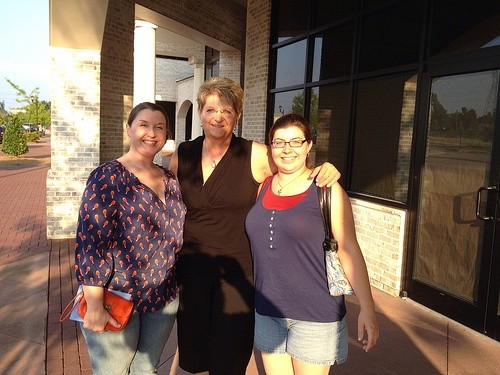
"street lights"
31,95,39,134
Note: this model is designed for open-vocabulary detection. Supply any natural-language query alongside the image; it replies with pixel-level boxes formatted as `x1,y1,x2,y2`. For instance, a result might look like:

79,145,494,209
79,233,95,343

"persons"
168,77,341,375
244,114,380,375
74,101,187,375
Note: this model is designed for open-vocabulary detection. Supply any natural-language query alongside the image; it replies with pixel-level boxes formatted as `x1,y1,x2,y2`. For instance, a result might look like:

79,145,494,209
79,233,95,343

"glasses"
200,106,238,119
270,137,310,148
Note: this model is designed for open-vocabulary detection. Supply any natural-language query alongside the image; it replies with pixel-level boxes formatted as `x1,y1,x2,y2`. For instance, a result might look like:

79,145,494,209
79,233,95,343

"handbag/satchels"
57,286,136,334
316,176,355,299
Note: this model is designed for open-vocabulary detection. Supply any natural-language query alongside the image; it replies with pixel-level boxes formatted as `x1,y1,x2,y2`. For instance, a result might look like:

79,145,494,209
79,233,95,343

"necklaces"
276,168,307,195
204,138,228,168
